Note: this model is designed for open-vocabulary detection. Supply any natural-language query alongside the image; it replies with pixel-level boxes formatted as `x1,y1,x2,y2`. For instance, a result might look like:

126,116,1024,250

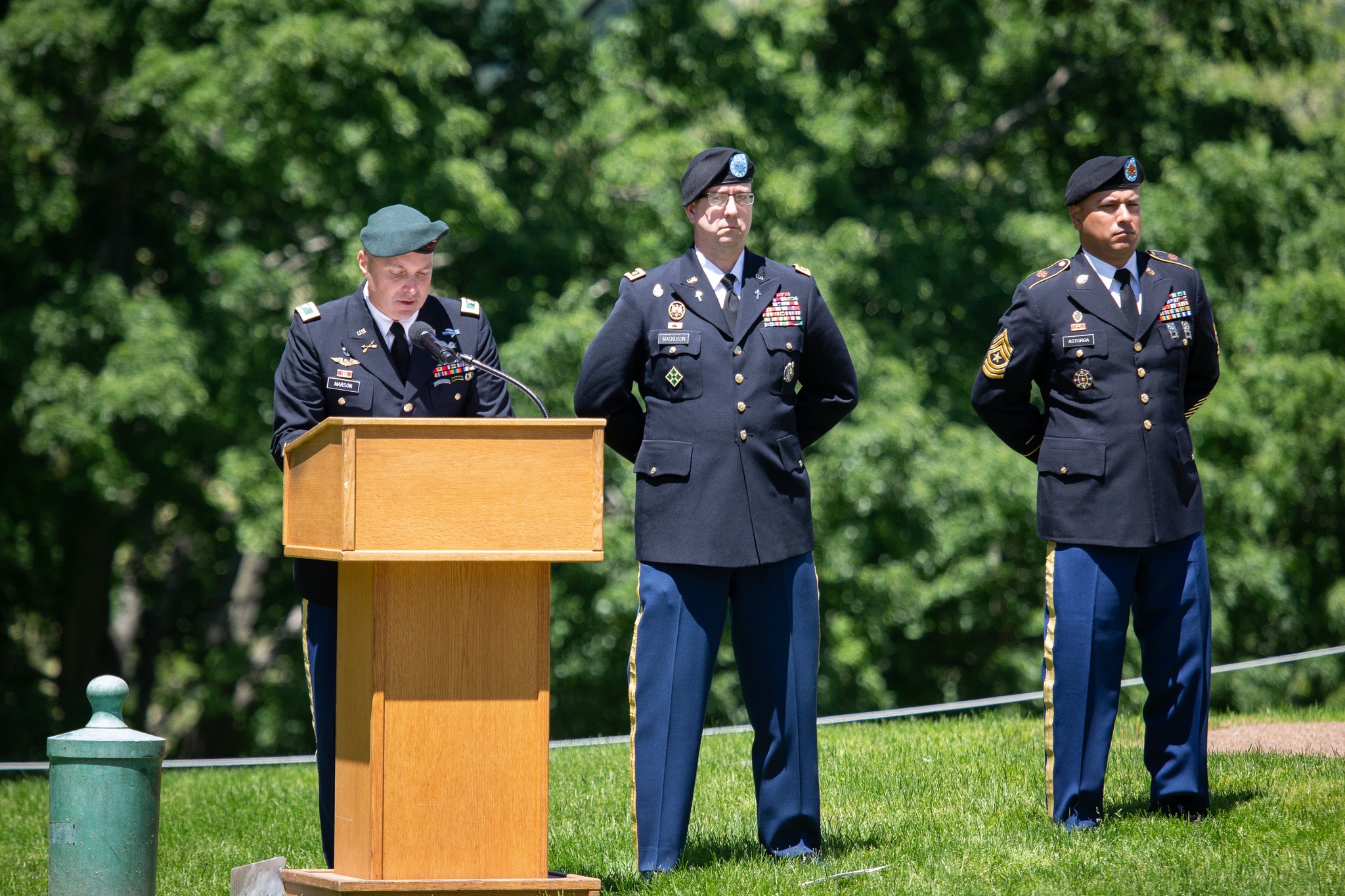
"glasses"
697,191,755,206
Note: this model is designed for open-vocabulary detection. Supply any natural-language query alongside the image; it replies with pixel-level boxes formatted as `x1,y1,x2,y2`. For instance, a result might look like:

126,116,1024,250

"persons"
970,156,1222,834
270,204,516,871
576,148,861,878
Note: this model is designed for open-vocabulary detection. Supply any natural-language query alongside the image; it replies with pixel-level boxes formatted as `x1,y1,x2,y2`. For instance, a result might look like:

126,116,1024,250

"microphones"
408,321,456,364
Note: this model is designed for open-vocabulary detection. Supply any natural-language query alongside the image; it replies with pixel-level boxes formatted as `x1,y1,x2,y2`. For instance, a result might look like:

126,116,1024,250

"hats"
1064,156,1144,207
359,204,450,257
679,147,755,206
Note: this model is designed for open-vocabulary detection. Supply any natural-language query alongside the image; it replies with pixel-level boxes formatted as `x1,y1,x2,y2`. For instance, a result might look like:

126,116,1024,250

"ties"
720,274,739,338
389,322,409,389
1114,268,1139,332
